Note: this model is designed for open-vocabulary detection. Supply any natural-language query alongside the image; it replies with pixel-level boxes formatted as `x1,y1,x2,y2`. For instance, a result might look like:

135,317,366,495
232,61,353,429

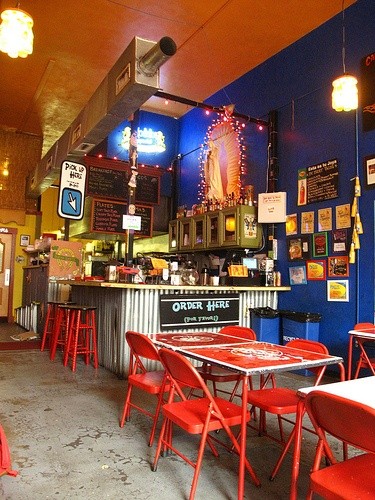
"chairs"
119,326,375,500
354,323,375,379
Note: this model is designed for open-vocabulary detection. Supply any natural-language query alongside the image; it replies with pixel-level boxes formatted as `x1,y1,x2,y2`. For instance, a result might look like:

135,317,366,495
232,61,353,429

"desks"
142,331,255,398
290,376,375,500
176,342,345,500
348,328,375,380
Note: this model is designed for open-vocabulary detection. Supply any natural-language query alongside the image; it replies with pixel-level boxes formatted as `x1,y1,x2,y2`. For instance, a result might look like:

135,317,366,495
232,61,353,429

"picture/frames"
287,228,349,302
362,154,375,190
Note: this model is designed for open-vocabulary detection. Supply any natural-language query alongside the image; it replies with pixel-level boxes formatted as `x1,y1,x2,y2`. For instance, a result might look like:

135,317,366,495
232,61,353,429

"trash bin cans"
281,310,322,346
249,306,280,346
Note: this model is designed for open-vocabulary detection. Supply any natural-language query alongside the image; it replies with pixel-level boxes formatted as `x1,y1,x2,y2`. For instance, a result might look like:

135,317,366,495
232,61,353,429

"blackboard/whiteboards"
85,164,161,206
159,293,240,330
89,199,154,238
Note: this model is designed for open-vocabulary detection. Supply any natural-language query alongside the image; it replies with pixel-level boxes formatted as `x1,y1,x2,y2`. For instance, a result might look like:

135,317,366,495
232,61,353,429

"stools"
41,301,98,372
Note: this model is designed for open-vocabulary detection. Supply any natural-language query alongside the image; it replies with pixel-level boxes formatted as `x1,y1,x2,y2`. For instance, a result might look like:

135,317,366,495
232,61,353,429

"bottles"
349,177,363,263
300,181,305,203
176,188,257,219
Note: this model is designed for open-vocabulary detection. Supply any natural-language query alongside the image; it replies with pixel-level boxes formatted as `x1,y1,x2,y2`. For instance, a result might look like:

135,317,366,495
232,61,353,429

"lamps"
1,0,34,58
331,0,359,112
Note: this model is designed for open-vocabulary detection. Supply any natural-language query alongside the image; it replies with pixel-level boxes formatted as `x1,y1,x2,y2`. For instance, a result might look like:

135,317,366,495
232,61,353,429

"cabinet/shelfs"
169,205,262,252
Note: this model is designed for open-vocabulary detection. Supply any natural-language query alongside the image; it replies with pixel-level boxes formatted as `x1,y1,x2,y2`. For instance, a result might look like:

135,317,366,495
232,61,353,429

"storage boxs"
226,276,249,285
